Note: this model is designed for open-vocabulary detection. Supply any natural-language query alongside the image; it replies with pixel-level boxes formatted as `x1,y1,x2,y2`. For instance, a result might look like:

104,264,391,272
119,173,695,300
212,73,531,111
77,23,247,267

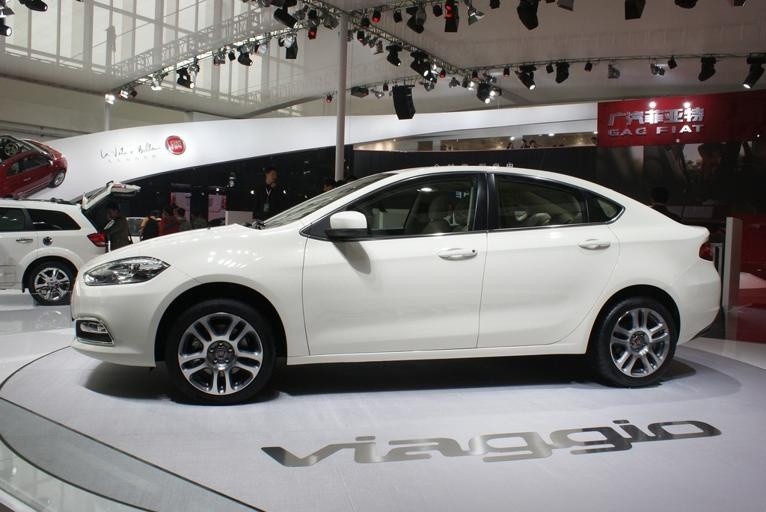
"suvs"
0,178,141,306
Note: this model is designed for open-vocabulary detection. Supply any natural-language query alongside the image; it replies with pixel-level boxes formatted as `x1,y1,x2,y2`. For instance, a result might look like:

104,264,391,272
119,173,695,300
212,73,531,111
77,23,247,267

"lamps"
0,0,48,37
98,0,766,122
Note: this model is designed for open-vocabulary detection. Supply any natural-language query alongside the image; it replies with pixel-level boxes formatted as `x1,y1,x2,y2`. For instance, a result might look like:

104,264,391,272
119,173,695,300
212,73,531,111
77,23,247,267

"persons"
690,142,726,195
140,206,208,240
103,202,129,251
323,174,389,226
252,167,287,222
650,183,680,222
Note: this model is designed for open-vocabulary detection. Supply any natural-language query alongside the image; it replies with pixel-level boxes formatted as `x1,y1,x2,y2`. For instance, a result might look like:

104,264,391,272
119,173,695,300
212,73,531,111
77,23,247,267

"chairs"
420,194,452,234
453,197,472,231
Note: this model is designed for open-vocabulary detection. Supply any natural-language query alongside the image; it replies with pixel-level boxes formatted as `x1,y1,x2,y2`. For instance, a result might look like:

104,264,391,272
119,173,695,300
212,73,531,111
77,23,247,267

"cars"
0,129,69,200
65,160,730,409
